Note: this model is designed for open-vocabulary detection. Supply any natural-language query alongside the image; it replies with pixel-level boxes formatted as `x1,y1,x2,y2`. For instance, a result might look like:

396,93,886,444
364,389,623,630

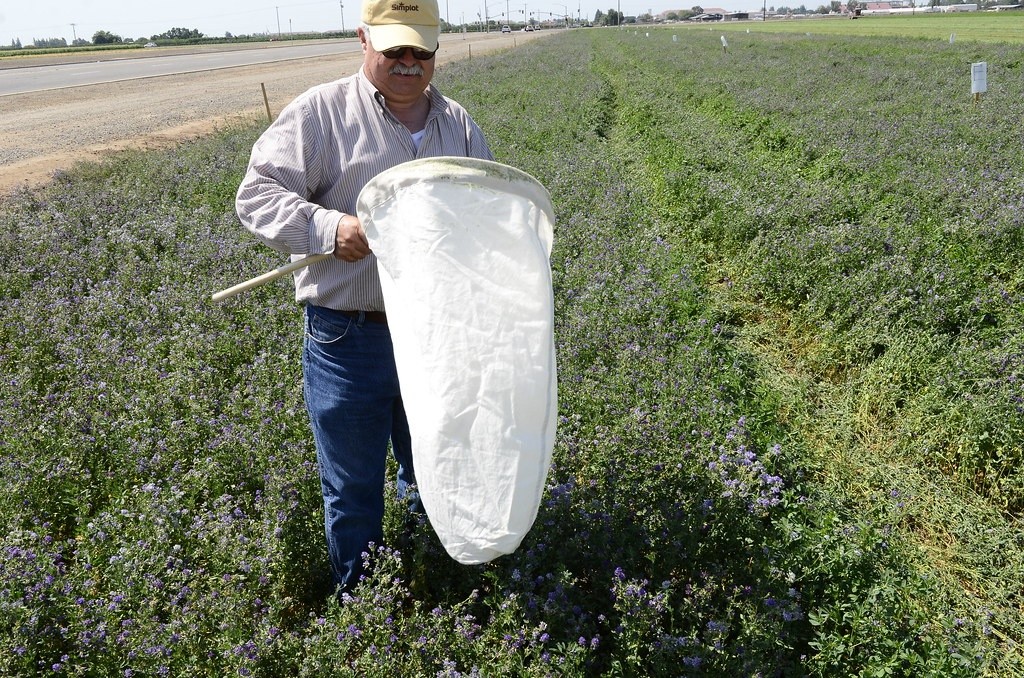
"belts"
334,309,387,325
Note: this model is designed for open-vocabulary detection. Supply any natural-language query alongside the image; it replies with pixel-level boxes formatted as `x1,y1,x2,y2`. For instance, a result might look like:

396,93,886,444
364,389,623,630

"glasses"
381,41,439,60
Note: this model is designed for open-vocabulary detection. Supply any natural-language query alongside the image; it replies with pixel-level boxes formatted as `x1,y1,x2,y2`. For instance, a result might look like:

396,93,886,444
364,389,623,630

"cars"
535,25,541,31
526,25,534,32
501,25,511,33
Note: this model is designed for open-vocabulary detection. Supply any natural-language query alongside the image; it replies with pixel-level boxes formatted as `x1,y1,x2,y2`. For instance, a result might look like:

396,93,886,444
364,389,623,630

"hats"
361,0,441,52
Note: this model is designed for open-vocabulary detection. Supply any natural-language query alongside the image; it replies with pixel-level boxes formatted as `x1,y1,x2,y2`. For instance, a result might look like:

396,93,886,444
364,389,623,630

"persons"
234,1,500,602
